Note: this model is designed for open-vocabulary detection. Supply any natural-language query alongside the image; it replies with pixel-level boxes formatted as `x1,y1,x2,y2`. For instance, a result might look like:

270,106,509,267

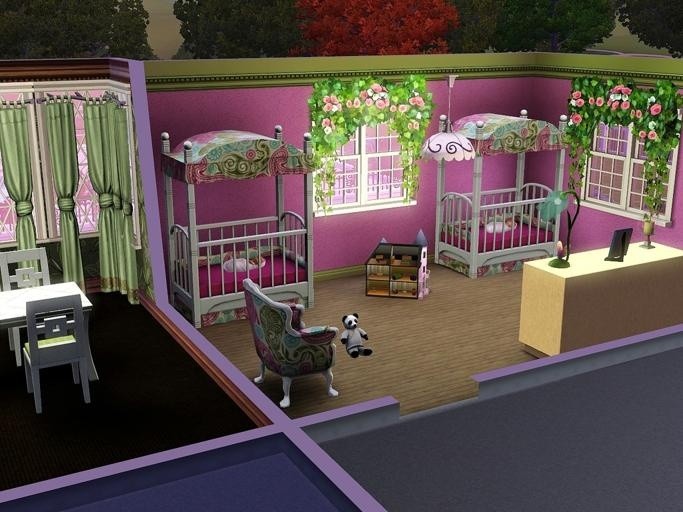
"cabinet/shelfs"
364,242,428,300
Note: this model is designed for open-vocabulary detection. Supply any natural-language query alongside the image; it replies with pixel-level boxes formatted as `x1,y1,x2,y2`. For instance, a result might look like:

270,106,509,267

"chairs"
242,278,339,410
0,247,92,414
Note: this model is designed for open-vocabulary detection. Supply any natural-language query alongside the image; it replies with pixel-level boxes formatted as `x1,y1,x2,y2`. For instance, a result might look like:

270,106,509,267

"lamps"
419,75,475,163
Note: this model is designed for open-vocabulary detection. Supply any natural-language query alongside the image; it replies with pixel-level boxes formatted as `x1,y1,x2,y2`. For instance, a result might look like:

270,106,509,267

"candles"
643,219,654,234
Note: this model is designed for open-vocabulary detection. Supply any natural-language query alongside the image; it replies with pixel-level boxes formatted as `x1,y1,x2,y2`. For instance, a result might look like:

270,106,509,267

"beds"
435,213,560,279
169,245,314,329
